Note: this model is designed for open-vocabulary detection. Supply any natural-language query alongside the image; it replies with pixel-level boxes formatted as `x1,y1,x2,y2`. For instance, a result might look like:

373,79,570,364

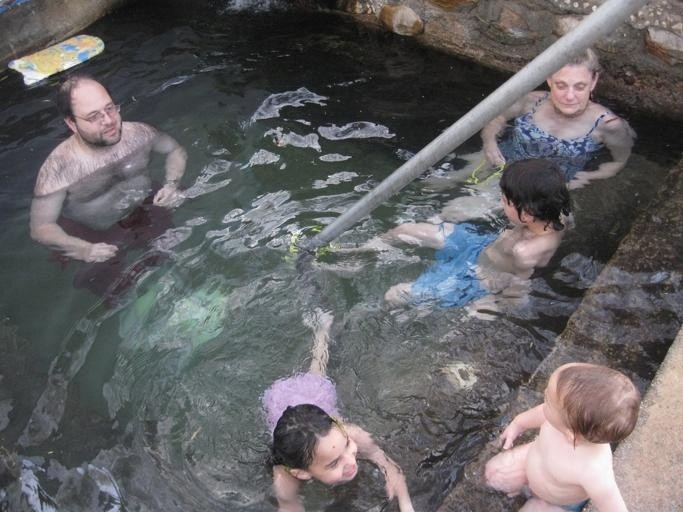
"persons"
27,73,189,262
484,361,641,512
259,306,417,512
363,159,576,324
423,46,636,224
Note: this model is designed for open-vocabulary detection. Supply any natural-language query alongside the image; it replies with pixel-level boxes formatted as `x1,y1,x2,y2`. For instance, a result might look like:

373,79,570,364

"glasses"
65,103,121,125
